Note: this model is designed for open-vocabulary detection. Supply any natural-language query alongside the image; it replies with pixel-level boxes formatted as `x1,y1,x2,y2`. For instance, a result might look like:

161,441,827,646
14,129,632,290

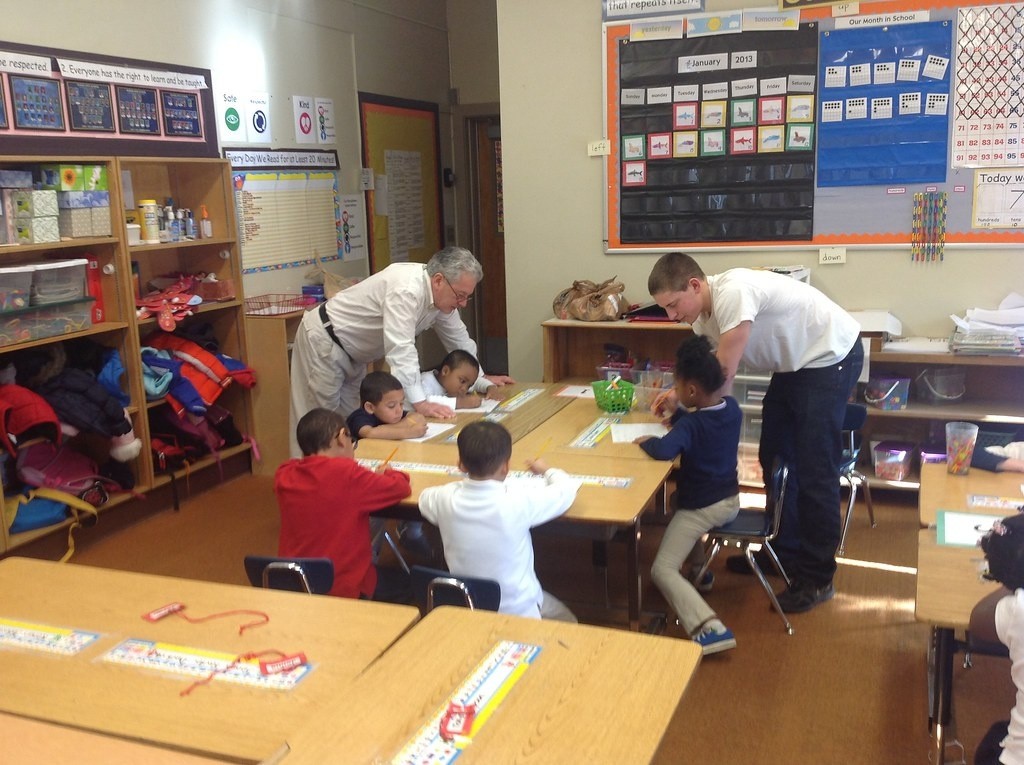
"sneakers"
691,624,738,656
691,570,714,592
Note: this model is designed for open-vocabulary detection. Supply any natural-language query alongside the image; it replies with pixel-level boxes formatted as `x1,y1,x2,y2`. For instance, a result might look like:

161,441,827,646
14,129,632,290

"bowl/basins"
127,224,141,244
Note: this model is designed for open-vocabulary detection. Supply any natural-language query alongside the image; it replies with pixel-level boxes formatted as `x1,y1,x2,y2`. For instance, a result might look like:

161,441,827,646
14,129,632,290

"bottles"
200,205,212,238
185,211,198,239
138,199,160,244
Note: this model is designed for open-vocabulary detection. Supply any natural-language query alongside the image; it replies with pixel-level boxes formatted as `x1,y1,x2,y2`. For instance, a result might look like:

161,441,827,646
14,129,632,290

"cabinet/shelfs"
245,300,373,474
116,157,255,509
840,347,1024,489
538,315,694,468
0,156,151,558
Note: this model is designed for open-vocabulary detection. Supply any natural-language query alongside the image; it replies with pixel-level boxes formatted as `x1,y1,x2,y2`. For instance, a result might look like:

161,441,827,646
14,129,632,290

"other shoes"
398,532,436,558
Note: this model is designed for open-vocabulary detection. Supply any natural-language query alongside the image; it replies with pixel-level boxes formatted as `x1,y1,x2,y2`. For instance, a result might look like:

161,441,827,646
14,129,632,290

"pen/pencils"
606,375,622,391
408,417,417,424
524,434,553,473
473,391,477,395
379,448,398,467
975,524,990,532
654,387,674,408
612,381,631,410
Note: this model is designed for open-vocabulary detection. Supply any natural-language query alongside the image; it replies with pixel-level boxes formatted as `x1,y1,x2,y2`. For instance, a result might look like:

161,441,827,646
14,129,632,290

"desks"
0,555,422,765
914,527,1024,765
312,372,688,630
267,605,706,765
921,458,1023,531
0,712,242,765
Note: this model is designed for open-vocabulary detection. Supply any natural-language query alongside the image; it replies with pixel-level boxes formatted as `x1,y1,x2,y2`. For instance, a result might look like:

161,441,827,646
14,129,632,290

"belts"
319,301,349,356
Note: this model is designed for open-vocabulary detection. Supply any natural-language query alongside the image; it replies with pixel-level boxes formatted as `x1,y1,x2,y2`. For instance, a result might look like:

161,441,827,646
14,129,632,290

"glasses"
432,271,474,302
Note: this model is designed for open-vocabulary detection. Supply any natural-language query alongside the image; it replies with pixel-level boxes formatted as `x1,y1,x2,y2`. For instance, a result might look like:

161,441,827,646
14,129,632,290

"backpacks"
0,400,243,534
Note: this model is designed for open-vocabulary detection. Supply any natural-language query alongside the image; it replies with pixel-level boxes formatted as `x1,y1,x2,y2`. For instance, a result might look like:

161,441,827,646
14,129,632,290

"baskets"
591,379,634,412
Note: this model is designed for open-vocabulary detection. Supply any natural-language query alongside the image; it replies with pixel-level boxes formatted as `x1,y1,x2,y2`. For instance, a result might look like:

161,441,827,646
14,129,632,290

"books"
747,385,767,405
950,314,1024,354
750,416,763,423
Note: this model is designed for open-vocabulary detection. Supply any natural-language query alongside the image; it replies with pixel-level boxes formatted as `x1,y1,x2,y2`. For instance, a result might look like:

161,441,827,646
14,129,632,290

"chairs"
836,404,877,555
244,556,333,595
647,464,790,636
410,567,500,617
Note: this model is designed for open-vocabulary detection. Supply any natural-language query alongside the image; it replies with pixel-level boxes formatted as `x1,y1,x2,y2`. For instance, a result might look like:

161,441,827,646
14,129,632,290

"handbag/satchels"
552,275,631,322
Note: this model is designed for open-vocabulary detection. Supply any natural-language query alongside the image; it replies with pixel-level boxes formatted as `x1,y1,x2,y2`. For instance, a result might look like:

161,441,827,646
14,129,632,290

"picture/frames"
782,123,815,150
730,126,756,155
158,86,203,139
729,100,756,126
621,134,646,160
63,77,117,133
0,73,9,129
673,130,697,158
672,102,699,131
753,124,785,156
699,100,726,127
647,132,671,158
756,95,786,124
784,96,814,123
622,160,647,187
113,83,162,136
7,72,66,133
698,129,725,157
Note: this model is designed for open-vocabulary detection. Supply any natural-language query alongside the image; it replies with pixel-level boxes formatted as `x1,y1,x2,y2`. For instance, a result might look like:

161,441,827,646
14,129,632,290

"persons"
417,421,579,624
646,251,864,616
288,246,517,459
974,513,1024,765
632,335,744,657
272,407,412,599
346,370,429,443
402,349,482,418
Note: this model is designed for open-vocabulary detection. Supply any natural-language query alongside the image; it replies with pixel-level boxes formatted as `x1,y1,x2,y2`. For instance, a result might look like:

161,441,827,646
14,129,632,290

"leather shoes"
727,550,793,577
771,576,834,612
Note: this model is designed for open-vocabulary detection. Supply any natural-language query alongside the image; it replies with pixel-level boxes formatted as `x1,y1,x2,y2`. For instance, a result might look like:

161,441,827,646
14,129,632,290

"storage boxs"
595,331,1017,489
0,165,111,348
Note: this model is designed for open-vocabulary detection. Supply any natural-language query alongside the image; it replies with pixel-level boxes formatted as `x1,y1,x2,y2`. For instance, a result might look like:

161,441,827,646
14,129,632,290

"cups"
945,422,979,475
